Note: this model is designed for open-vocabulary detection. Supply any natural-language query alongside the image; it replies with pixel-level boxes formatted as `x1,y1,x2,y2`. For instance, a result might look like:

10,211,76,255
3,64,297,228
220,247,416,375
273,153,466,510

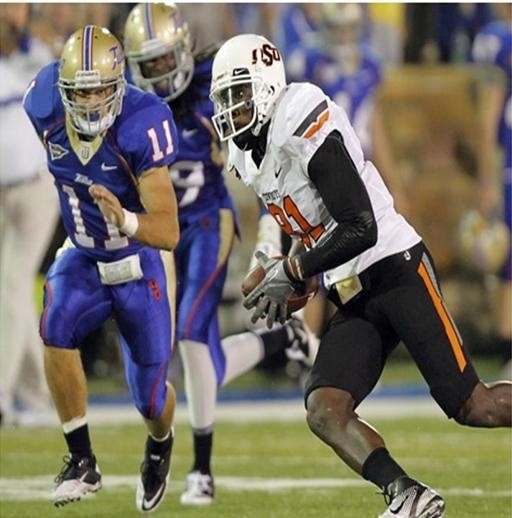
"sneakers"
379,475,445,518
135,426,174,512
54,455,101,507
180,471,214,505
285,316,321,367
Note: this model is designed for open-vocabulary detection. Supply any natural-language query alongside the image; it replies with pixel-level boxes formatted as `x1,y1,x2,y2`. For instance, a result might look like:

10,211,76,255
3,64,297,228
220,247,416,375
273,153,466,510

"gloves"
243,251,300,328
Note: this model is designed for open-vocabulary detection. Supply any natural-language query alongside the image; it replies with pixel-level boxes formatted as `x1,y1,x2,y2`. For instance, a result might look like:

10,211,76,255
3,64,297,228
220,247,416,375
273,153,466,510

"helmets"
209,34,287,143
58,25,125,136
124,2,194,101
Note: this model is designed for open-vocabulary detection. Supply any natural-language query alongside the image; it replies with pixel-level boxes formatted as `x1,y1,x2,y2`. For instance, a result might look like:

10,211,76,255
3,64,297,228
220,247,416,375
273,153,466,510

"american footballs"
242,255,319,311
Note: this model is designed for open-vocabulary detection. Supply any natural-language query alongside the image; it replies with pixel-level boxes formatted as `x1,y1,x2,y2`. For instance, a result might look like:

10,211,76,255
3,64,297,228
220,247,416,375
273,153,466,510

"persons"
116,2,319,509
20,22,181,512
2,2,510,430
208,32,511,518
